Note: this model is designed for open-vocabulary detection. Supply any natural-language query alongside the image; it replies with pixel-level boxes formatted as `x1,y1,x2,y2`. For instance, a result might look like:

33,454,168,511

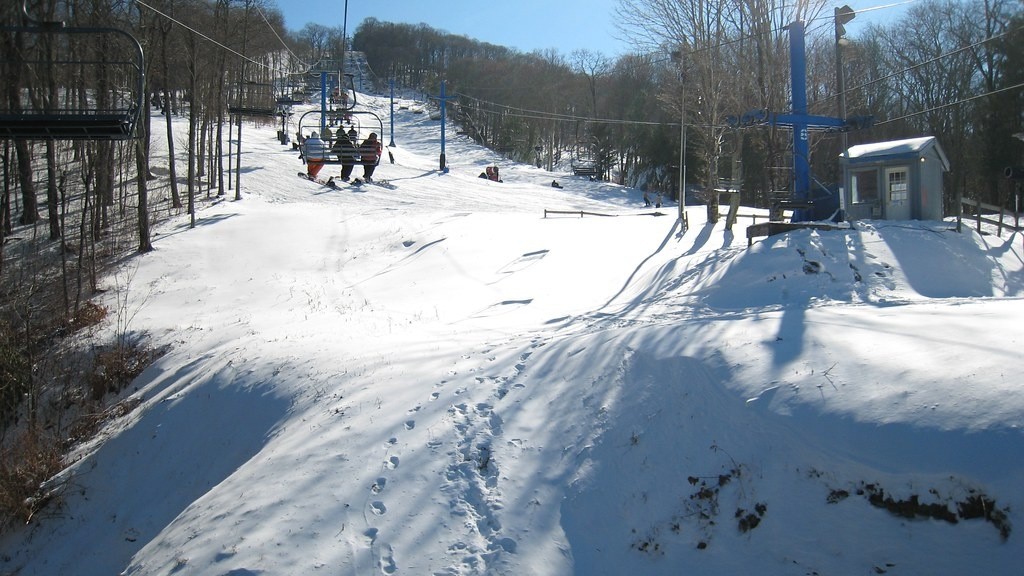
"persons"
644,193,651,206
296,133,305,143
552,180,563,188
331,133,355,180
320,125,333,147
348,126,357,144
344,109,350,124
330,109,335,124
477,165,498,181
336,125,345,136
335,108,343,125
330,93,348,104
360,133,380,182
657,195,662,206
303,131,326,181
